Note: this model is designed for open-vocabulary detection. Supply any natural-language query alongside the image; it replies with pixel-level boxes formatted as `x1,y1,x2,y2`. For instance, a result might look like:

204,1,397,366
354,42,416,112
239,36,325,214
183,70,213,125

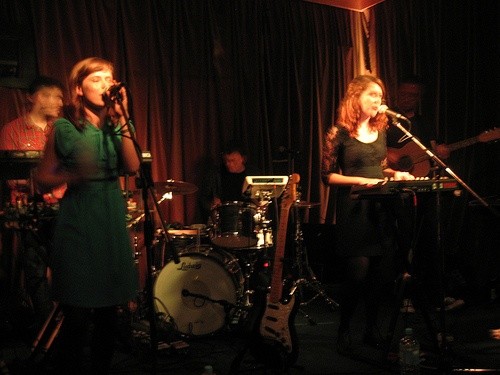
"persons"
319,75,449,361
0,57,141,375
206,144,258,209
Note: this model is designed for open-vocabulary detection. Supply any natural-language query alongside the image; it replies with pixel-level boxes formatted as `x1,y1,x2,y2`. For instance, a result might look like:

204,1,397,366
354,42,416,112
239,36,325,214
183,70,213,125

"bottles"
399,328,420,375
203,366,216,375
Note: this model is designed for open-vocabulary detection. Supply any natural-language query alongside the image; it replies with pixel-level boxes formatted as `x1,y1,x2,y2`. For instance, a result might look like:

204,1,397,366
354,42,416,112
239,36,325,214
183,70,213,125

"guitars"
252,173,301,366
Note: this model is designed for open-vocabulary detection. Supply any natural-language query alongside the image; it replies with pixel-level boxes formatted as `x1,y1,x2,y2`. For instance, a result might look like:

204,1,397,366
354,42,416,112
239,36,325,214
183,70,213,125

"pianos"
0,149,152,375
350,178,468,375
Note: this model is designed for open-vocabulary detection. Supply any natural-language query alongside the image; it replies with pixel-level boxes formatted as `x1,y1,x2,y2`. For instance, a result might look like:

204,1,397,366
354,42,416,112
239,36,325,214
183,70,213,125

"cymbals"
153,179,199,195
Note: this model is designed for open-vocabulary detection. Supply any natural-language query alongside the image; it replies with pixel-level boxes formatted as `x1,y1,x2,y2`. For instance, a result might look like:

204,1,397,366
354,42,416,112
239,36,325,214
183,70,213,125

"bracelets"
122,127,130,134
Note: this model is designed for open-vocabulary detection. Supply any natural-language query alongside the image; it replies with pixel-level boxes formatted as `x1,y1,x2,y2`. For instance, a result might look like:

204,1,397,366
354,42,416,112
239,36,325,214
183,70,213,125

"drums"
206,199,266,249
156,229,211,252
152,244,246,342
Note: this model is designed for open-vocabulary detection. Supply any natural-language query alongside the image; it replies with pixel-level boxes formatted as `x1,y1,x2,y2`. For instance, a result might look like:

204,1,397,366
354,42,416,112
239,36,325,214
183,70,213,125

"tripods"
283,206,340,308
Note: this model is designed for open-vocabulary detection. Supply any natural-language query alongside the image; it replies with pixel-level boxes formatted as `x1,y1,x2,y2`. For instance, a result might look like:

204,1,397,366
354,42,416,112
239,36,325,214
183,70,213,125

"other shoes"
337,327,352,355
362,322,384,347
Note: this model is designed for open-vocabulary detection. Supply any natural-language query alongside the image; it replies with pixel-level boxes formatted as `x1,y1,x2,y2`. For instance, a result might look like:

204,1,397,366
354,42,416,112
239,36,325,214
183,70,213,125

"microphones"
182,289,189,297
378,104,407,120
105,82,124,97
278,146,302,155
